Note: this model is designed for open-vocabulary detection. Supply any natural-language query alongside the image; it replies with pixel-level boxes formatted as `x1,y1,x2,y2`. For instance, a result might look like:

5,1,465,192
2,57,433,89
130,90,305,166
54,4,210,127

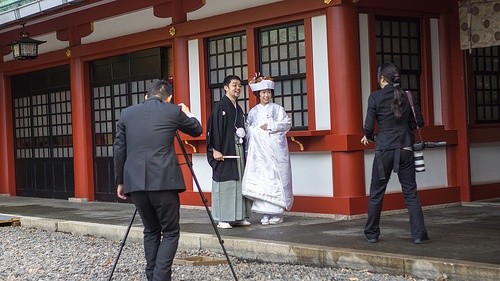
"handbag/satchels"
413,143,426,173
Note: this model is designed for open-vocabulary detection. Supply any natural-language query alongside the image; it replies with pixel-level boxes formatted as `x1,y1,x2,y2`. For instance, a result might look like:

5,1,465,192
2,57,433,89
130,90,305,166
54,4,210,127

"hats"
248,72,276,91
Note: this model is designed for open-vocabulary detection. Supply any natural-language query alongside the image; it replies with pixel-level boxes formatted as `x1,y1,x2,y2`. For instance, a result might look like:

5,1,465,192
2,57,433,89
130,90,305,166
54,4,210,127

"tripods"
108,127,238,281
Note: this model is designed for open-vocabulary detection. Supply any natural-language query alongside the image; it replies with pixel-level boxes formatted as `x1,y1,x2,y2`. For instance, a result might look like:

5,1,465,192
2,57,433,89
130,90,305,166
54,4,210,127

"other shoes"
217,221,232,229
414,237,430,244
234,220,251,226
366,236,378,242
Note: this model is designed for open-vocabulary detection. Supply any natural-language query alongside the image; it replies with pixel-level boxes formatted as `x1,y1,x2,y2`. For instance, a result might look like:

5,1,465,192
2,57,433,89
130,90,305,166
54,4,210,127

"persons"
361,62,431,244
206,75,252,228
241,75,294,224
113,80,203,281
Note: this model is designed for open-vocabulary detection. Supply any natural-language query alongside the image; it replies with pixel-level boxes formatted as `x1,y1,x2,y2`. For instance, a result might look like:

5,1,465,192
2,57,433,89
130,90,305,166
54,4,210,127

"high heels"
260,214,271,225
269,214,284,225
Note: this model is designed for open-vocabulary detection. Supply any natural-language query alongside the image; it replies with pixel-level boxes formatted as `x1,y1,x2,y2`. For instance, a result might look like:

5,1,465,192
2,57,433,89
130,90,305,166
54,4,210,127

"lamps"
8,20,47,60
